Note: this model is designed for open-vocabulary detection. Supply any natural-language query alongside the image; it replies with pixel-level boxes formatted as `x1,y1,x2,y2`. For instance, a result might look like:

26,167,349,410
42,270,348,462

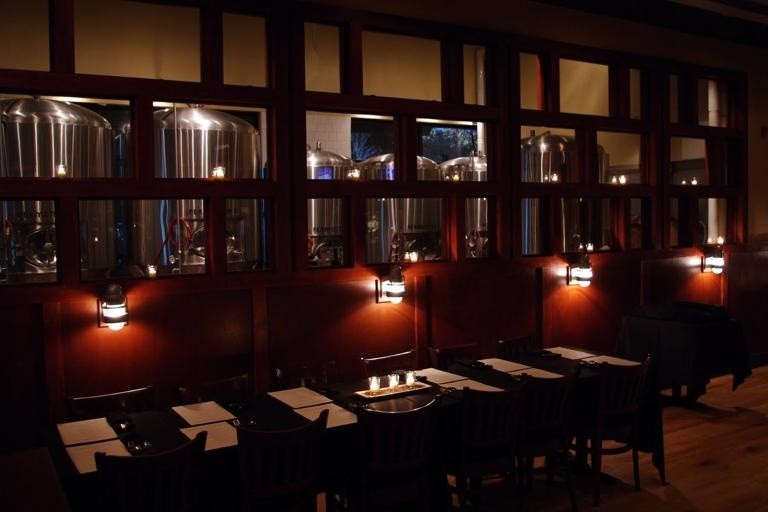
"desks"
619,311,744,404
